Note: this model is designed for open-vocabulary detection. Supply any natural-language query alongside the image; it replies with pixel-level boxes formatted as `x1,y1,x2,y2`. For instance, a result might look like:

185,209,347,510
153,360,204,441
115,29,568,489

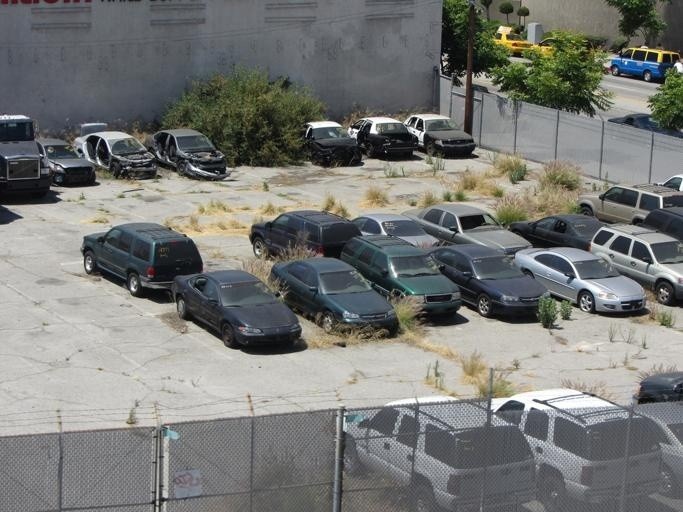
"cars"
267,255,399,338
672,59,683,78
300,119,360,165
350,116,418,156
1,111,235,194
405,111,477,158
621,372,681,501
526,36,557,62
491,25,531,55
170,268,301,348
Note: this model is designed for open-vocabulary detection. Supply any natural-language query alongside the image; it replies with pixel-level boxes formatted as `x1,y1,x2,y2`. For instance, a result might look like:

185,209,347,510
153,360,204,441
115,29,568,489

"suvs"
79,221,204,300
486,386,665,510
248,208,359,262
336,392,537,512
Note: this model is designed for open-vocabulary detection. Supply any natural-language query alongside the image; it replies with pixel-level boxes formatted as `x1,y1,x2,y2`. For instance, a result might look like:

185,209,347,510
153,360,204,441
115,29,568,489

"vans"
610,45,681,83
